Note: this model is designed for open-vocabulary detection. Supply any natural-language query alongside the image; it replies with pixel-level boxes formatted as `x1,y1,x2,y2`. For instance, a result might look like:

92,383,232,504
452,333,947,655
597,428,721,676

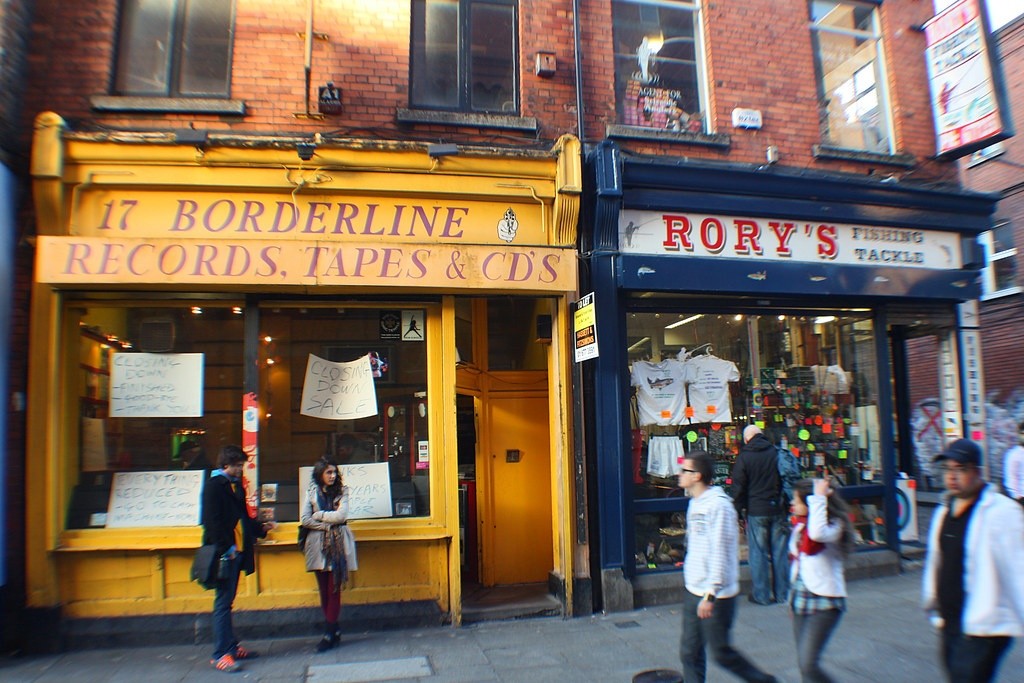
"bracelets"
702,593,716,603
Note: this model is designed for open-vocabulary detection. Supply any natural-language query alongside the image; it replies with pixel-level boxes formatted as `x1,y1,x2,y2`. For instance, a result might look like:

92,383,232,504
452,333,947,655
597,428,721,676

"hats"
933,439,985,467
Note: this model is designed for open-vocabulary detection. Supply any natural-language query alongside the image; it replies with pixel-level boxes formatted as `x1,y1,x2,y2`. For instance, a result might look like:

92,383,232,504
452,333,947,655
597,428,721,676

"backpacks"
769,442,807,502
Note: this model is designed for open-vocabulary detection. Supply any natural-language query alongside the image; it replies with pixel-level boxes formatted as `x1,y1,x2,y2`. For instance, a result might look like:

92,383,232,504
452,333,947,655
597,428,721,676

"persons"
201,444,273,673
678,451,779,683
923,438,1024,683
725,423,789,605
786,477,852,682
1003,423,1024,508
301,456,358,652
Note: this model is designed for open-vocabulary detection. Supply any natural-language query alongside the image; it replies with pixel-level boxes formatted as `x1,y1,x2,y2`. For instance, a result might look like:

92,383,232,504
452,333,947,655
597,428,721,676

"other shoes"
311,631,341,652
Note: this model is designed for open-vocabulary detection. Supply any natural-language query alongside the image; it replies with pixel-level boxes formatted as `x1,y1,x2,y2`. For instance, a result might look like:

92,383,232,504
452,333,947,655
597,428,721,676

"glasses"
681,468,696,474
943,462,973,472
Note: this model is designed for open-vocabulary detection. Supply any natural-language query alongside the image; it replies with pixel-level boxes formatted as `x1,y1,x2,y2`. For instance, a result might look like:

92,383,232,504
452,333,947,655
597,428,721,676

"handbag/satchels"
189,544,229,596
296,522,308,554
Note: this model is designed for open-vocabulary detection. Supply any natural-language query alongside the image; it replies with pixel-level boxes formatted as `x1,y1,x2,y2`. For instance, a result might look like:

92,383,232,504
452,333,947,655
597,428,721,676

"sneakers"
232,646,259,659
210,653,245,673
